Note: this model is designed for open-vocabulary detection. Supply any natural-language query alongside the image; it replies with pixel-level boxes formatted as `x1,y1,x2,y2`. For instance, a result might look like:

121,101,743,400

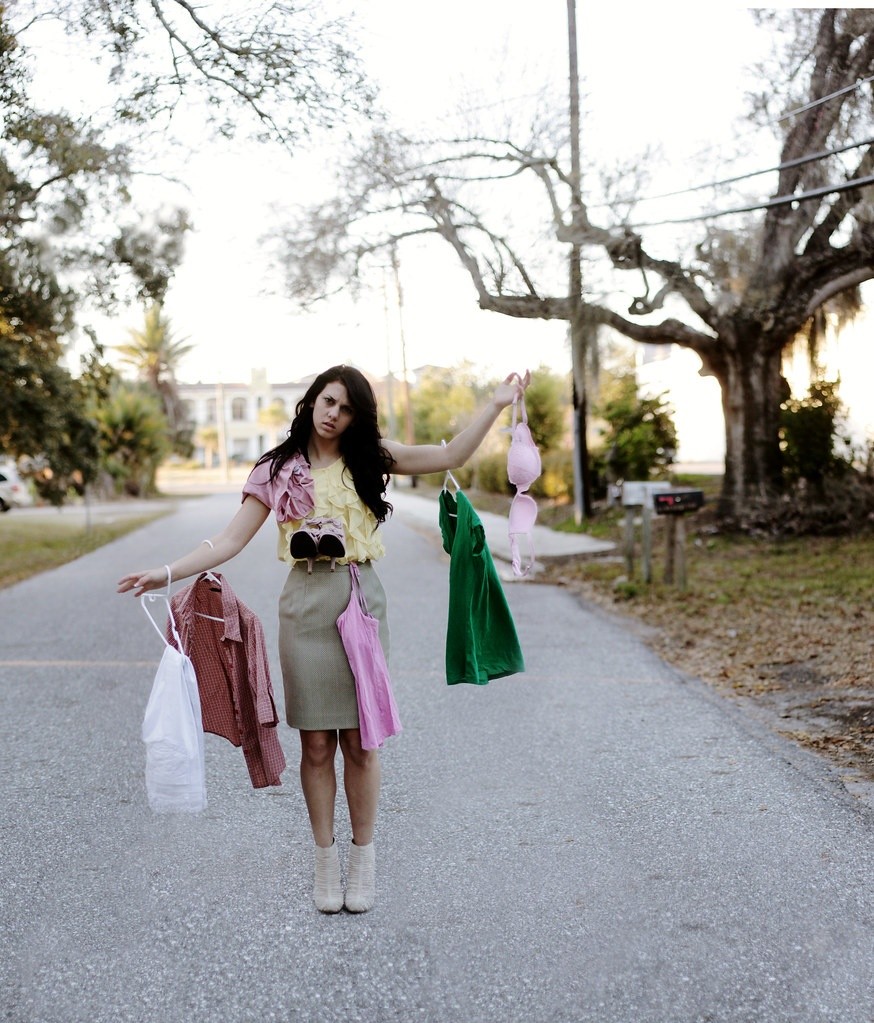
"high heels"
318,519,345,572
290,517,319,575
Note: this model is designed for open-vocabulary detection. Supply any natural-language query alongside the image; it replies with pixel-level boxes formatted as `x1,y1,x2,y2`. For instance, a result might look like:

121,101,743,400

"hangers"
141,564,183,656
193,540,225,622
438,440,460,517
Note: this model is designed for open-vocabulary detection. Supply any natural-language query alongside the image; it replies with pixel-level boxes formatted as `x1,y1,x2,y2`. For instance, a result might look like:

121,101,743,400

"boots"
345,838,375,913
314,837,344,914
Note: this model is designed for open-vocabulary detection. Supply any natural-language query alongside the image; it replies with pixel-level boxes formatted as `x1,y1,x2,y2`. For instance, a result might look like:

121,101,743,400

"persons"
118,366,530,914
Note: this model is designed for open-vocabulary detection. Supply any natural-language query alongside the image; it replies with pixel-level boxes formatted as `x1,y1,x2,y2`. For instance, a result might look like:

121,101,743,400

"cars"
0,465,33,511
